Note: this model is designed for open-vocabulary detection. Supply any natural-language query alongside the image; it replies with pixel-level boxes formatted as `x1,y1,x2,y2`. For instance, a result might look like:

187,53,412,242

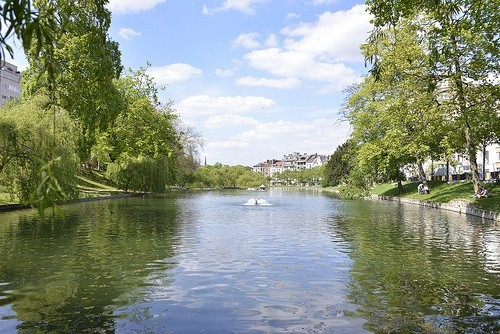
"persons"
470,182,489,198
417,180,429,195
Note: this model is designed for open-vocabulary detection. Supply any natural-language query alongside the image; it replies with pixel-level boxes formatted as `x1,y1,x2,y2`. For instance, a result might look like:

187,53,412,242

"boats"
249,198,266,205
